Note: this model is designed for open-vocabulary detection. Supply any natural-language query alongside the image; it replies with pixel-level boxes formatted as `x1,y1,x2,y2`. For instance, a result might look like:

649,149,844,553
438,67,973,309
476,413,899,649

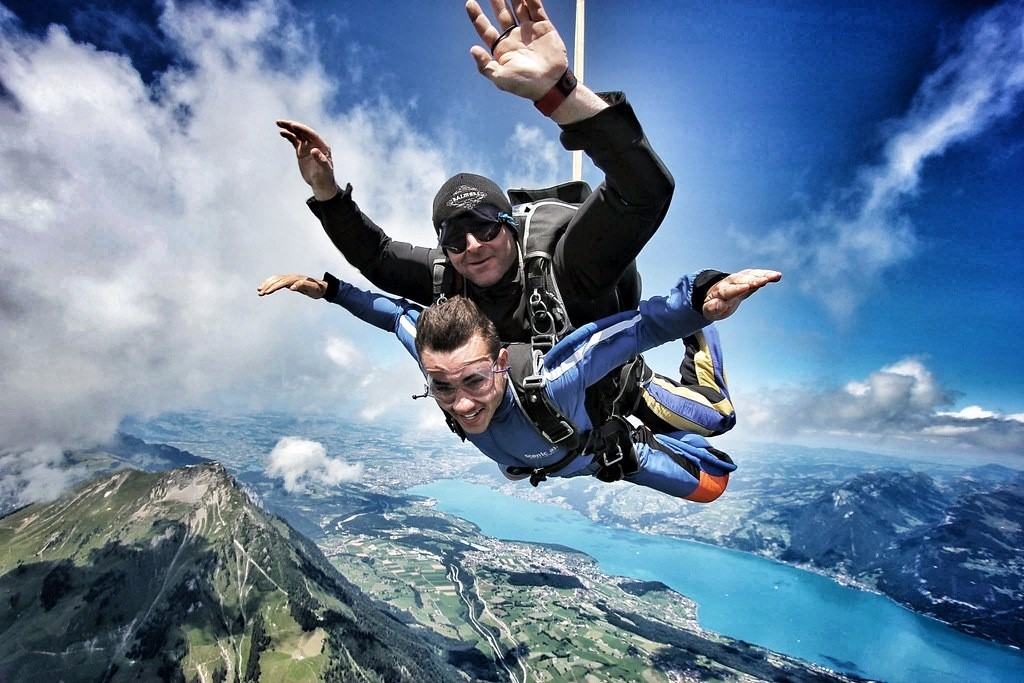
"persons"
275,0,737,438
256,269,782,504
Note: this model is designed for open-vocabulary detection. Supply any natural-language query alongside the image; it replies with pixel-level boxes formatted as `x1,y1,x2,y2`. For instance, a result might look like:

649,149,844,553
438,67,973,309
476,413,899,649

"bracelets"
534,66,577,117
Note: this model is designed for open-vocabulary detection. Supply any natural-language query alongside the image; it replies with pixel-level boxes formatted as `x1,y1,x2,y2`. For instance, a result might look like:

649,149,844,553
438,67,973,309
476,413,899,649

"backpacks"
433,181,642,340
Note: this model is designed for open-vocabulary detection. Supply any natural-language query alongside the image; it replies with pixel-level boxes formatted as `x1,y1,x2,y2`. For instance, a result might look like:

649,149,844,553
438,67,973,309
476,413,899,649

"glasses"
428,359,497,403
445,222,501,254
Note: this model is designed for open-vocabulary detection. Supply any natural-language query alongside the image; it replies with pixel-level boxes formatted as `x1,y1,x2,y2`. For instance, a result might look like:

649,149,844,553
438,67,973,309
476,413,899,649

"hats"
432,173,512,246
